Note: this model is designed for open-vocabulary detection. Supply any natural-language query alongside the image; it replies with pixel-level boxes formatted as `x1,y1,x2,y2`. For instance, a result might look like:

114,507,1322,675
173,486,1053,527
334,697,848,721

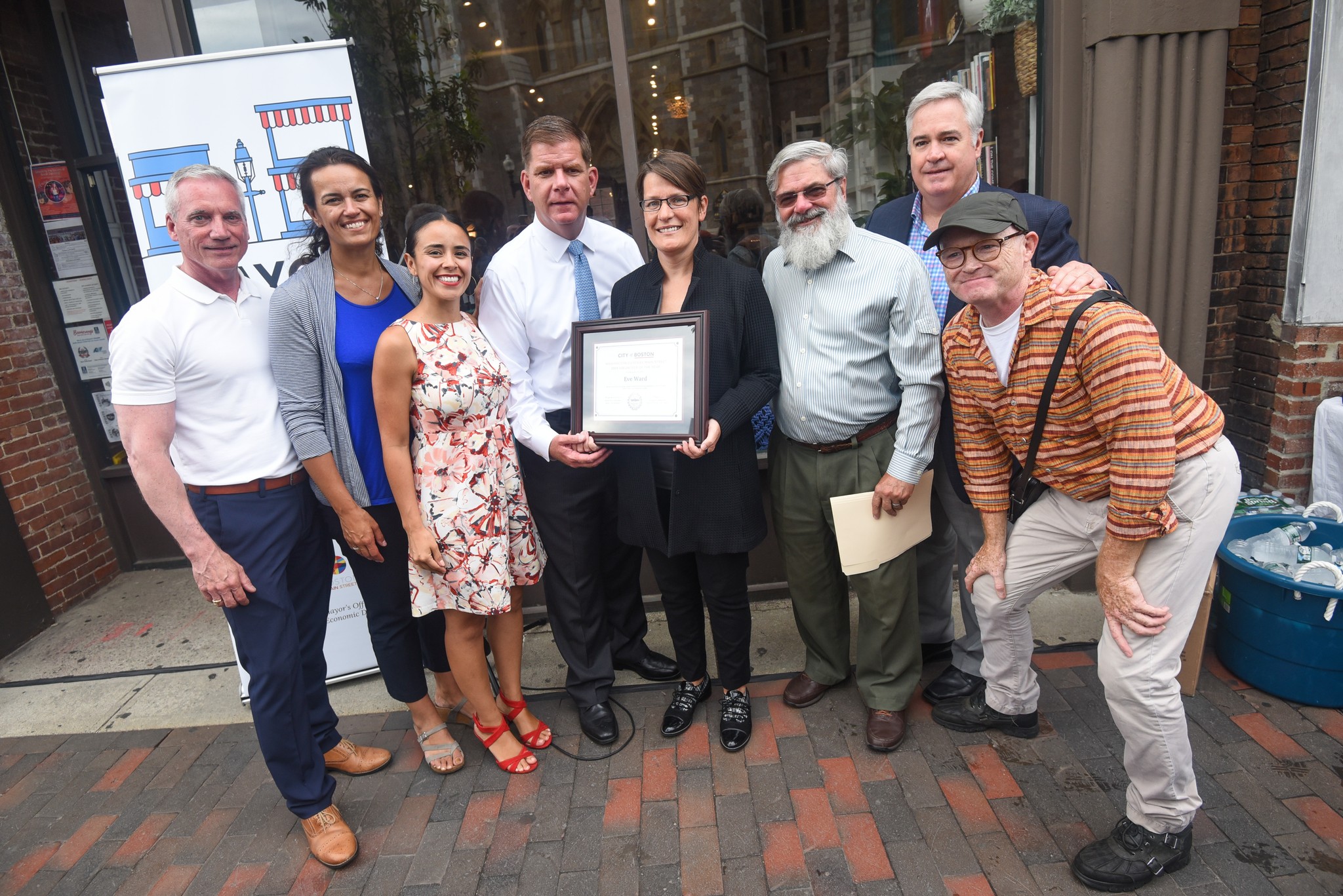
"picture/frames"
569,308,714,450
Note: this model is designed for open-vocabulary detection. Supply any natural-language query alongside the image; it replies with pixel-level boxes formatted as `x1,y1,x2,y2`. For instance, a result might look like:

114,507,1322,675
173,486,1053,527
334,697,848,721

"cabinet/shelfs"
789,21,1034,223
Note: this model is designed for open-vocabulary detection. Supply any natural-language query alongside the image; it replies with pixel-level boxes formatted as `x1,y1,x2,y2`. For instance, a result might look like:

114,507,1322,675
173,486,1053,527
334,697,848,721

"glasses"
639,192,697,212
773,178,841,209
935,229,1029,270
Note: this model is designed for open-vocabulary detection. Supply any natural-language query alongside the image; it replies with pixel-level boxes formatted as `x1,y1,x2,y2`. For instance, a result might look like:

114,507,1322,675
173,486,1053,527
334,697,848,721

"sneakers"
1069,816,1194,892
932,687,1040,739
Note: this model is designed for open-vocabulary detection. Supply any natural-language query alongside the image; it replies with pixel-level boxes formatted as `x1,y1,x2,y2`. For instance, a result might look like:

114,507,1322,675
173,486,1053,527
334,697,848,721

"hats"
922,191,1029,253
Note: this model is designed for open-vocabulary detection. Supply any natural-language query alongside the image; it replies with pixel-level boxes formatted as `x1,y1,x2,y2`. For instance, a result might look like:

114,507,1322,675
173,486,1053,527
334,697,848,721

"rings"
212,599,221,606
352,545,359,550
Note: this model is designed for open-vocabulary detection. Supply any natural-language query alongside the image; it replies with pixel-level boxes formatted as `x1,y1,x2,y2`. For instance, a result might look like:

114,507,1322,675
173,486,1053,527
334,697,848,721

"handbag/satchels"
1008,467,1044,524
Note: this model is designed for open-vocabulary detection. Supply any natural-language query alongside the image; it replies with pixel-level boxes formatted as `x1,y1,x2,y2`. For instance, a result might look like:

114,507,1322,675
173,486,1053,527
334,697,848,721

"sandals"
412,721,465,773
496,687,553,749
471,710,538,774
433,694,477,726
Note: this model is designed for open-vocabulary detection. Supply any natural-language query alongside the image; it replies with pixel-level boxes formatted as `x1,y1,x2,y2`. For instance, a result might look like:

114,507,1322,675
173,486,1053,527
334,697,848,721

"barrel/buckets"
1215,501,1343,708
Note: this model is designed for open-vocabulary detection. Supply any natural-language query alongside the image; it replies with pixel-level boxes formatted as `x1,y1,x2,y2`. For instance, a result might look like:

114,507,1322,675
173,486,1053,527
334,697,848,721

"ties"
567,240,603,322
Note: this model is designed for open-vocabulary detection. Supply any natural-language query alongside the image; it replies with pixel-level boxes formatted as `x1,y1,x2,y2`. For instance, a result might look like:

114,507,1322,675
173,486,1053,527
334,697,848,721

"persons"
700,188,779,266
476,113,678,745
269,146,482,773
762,139,942,754
864,83,1126,705
920,191,1242,892
109,164,392,869
371,202,554,773
567,149,782,753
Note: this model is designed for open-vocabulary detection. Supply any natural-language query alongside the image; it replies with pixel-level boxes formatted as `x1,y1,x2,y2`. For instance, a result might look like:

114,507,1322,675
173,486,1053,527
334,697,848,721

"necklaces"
332,261,383,303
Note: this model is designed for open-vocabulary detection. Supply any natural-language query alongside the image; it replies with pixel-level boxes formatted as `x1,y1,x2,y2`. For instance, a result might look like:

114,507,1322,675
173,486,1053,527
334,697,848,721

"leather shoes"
613,647,682,681
325,738,394,777
920,639,957,664
660,671,711,737
866,704,907,752
922,664,984,705
301,800,360,869
718,687,753,750
782,670,830,706
580,701,620,744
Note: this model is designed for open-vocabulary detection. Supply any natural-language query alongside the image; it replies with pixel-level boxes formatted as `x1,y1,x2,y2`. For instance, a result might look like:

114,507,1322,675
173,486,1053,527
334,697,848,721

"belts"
186,470,310,494
806,410,898,453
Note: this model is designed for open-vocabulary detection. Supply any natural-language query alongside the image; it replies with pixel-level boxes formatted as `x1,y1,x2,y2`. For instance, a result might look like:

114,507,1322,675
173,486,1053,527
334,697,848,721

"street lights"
502,154,518,199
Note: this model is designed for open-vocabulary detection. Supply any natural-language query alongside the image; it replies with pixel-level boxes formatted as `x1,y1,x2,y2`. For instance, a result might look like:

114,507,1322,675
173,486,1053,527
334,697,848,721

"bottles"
1245,521,1317,550
1251,540,1337,566
1317,543,1333,555
1330,549,1343,561
1288,562,1343,585
1232,489,1269,518
1227,539,1252,562
1271,491,1316,516
1262,561,1292,578
